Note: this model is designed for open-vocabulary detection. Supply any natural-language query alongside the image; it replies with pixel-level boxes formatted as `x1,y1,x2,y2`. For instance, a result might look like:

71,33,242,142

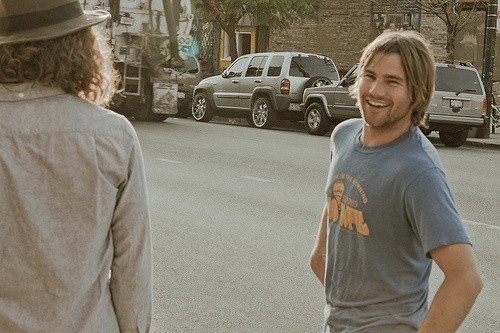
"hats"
0,0,111,44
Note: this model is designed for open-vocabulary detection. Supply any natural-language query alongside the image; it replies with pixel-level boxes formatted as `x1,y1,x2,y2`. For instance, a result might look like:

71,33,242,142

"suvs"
191,52,341,130
299,57,487,148
159,52,202,119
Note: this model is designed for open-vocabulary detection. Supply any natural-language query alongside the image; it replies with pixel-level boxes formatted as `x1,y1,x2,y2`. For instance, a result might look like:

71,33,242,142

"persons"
0,0,155,333
309,31,484,333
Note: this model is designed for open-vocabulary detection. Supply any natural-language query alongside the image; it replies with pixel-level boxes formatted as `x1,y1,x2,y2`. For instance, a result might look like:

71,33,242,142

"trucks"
76,0,194,124
